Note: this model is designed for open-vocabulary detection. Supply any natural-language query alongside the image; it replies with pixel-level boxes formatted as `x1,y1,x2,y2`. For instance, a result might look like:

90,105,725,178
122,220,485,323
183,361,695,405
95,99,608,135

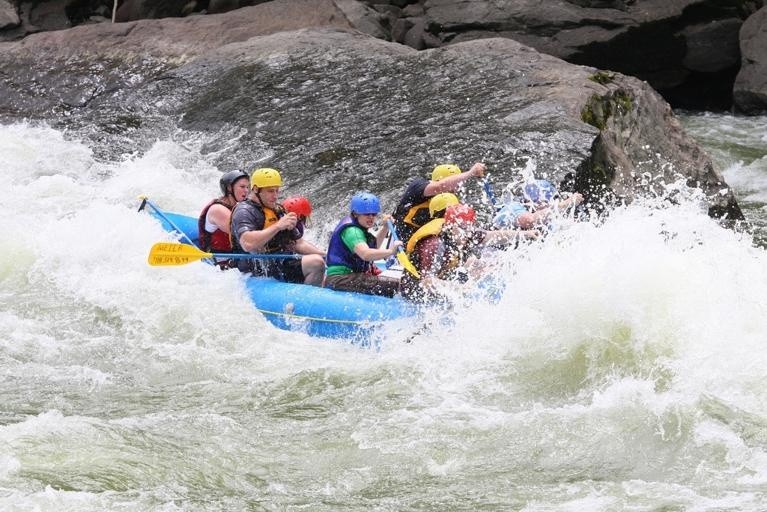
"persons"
200,170,327,287
326,162,584,301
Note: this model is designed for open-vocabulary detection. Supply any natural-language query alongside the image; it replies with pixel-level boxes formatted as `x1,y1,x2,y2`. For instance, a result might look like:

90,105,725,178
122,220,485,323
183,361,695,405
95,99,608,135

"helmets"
217,169,249,198
520,178,561,205
443,203,475,225
349,192,381,217
430,164,462,181
428,192,461,219
280,195,313,219
250,167,283,192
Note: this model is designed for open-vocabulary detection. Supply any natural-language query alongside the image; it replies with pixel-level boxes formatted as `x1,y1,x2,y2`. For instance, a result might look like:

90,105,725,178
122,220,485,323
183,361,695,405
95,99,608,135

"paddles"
381,213,420,279
148,244,302,267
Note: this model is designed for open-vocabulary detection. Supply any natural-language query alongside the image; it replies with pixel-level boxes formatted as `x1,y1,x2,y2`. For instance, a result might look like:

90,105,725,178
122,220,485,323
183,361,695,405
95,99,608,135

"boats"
149,209,500,347
